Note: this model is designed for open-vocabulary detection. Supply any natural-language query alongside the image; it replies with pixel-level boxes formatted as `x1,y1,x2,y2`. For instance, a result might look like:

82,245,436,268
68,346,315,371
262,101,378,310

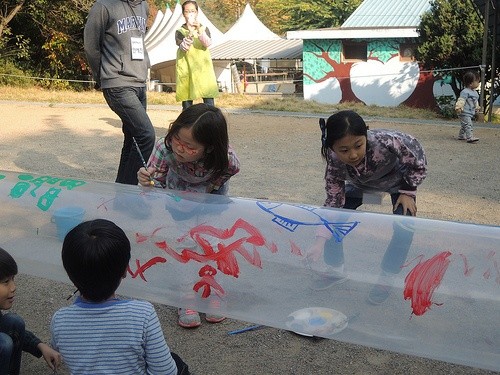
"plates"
285,306,348,337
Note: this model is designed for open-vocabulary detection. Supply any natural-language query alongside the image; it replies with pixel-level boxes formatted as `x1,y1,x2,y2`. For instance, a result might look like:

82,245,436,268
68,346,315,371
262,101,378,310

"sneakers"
310,266,348,290
368,273,397,304
206,299,225,321
177,292,201,327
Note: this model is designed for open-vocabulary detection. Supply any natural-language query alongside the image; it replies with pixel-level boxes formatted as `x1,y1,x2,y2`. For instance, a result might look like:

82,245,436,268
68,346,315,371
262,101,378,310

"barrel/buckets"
51,206,85,242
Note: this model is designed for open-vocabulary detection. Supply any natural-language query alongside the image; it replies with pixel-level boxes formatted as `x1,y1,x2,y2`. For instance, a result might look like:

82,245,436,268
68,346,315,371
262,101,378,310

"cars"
236,62,253,81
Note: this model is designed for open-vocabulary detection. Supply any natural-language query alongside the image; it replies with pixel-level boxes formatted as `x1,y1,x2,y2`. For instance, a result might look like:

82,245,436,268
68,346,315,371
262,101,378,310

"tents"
205,3,304,94
143,1,226,92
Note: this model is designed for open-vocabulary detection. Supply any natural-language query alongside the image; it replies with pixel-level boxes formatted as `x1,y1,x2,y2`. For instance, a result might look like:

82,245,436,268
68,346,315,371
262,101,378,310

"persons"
172,1,221,111
49,218,191,375
83,0,158,220
0,247,64,375
307,109,428,306
454,70,481,143
136,102,241,329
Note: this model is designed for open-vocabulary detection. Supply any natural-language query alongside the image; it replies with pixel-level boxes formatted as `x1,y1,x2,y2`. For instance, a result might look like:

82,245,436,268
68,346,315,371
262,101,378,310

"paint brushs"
67,290,78,301
314,310,360,338
227,316,294,335
132,136,155,186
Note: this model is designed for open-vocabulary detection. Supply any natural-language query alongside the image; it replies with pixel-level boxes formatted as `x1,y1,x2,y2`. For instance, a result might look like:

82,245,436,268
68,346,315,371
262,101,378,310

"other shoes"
457,136,467,140
467,137,480,143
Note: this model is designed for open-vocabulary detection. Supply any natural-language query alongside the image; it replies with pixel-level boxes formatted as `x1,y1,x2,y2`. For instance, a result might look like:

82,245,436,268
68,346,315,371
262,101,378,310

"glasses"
183,9,197,14
